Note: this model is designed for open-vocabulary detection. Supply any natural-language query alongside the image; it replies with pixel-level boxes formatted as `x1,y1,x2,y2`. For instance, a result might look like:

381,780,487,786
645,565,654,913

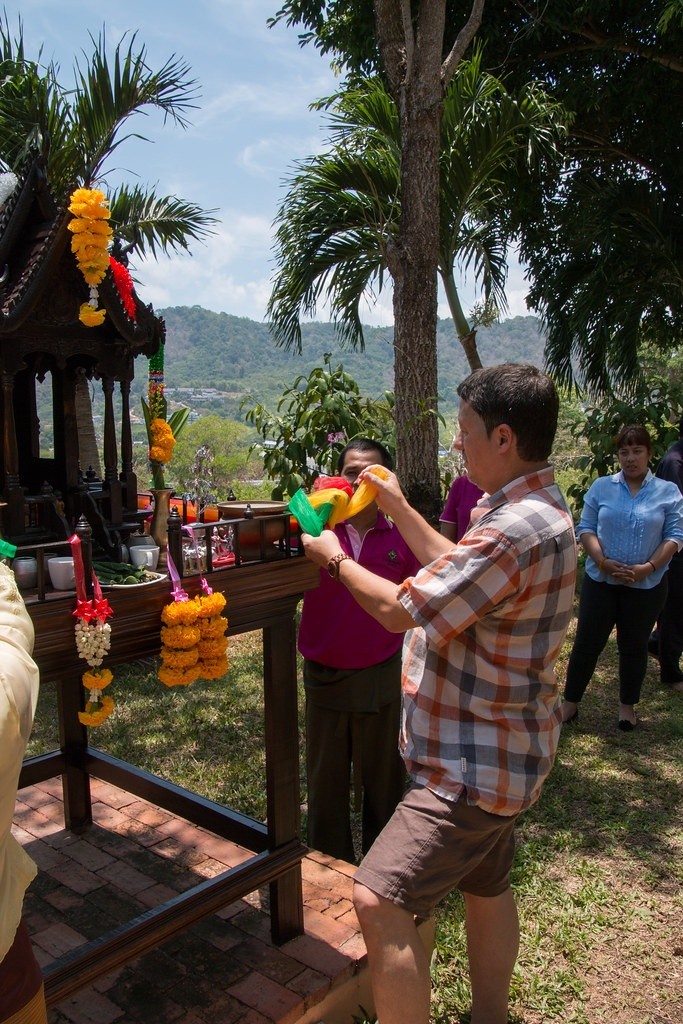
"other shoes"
619,711,636,730
563,709,578,723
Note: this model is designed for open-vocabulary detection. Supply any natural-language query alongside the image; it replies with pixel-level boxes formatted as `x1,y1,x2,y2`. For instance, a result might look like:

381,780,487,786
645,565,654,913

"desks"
11,553,322,1012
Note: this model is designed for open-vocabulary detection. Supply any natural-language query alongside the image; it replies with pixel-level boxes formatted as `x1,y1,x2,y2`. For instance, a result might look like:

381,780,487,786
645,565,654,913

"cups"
48,557,76,590
129,545,160,572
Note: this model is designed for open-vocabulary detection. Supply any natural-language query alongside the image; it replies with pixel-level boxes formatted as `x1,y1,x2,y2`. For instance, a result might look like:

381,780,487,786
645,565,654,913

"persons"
557,425,683,731
300,362,576,1024
0,561,48,1024
297,438,423,864
211,527,228,559
439,475,485,544
646,413,683,691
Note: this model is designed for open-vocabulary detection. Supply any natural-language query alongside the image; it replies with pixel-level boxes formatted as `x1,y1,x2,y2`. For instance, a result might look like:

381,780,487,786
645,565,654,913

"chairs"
82,494,141,553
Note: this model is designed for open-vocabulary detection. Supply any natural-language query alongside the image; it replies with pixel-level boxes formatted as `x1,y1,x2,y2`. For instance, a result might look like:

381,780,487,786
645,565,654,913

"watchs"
327,553,352,580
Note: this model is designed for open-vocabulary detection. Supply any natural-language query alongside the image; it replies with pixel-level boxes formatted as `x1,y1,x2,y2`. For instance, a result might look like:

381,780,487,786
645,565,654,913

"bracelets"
598,557,607,571
647,560,656,572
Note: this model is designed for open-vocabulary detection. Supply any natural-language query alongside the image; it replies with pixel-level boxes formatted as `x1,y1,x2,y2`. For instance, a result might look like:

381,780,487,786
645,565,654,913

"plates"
92,571,168,589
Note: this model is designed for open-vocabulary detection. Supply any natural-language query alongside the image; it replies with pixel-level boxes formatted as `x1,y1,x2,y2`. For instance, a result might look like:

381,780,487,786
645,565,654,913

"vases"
147,487,174,548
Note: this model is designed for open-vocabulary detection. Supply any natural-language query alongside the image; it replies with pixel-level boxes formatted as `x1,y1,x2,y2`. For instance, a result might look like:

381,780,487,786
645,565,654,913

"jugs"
113,543,129,563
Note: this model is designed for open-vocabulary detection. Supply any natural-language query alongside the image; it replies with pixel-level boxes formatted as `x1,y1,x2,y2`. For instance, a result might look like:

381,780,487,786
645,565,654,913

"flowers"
140,379,190,490
